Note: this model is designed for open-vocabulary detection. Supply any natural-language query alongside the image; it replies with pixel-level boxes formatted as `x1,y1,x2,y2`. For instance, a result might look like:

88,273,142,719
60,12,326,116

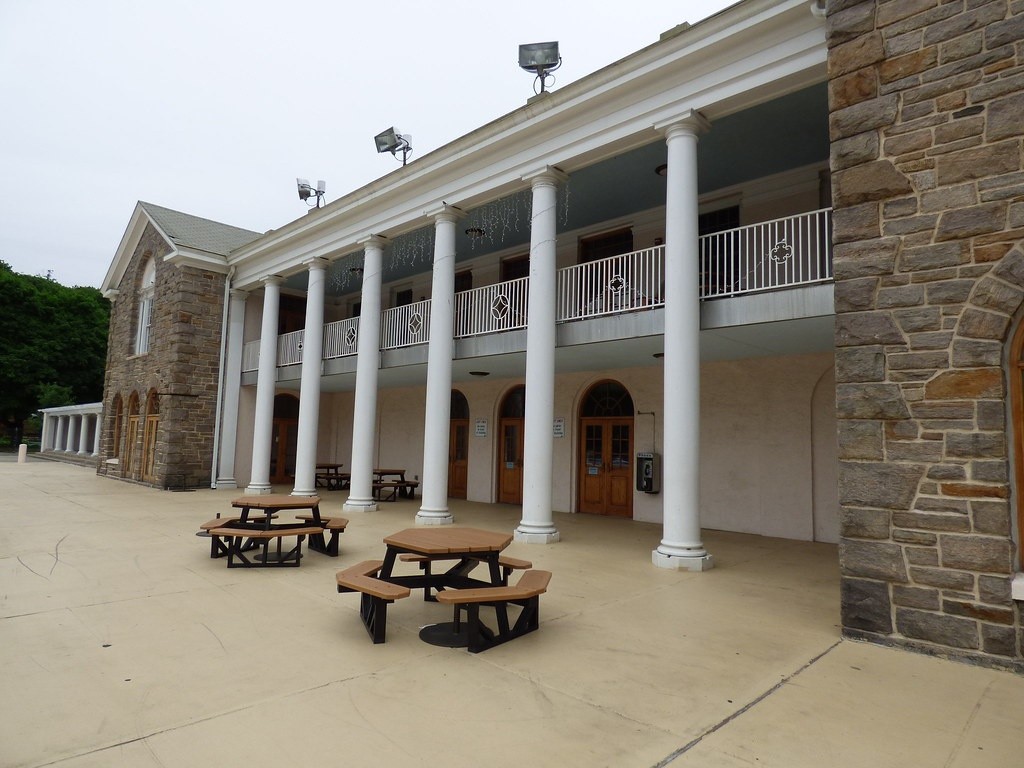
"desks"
379,526,514,645
373,468,407,499
315,463,343,489
231,496,326,560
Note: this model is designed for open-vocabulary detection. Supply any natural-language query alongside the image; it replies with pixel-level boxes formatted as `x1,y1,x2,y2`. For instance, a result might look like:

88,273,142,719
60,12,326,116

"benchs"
208,526,324,568
393,480,420,499
326,475,351,490
372,479,385,497
398,554,533,603
316,472,351,487
372,483,406,502
196,514,279,558
336,560,411,645
295,515,349,557
435,570,552,654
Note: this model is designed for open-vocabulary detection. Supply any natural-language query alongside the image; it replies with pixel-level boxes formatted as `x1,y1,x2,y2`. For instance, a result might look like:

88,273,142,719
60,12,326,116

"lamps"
654,353,664,359
297,177,326,213
465,228,486,239
656,164,667,177
374,126,413,167
469,371,490,377
517,41,563,105
349,267,363,274
